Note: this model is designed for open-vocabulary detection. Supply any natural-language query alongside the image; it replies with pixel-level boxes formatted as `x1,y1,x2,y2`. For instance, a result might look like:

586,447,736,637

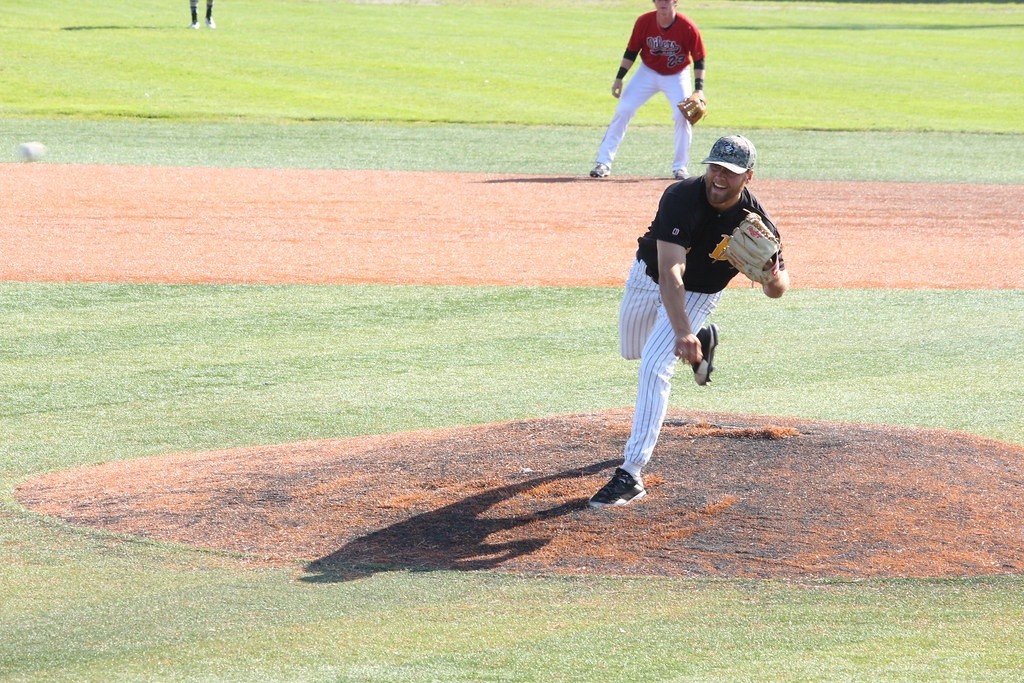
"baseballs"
19,141,49,162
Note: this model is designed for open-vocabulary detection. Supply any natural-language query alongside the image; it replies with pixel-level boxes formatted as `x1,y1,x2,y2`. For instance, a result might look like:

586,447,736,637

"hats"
701,134,756,174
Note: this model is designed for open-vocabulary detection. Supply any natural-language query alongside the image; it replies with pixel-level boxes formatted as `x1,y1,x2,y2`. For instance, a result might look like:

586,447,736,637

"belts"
636,250,659,285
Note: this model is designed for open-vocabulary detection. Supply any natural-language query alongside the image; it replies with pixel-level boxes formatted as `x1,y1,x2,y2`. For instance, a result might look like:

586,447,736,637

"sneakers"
692,325,718,385
673,169,690,180
590,162,610,177
589,466,646,510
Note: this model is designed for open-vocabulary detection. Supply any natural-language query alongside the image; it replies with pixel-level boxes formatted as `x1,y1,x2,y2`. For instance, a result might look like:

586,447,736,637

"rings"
680,351,683,353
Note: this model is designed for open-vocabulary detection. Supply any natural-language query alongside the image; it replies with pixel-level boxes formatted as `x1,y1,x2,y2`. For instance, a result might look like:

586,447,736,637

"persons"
587,133,790,510
589,0,708,181
187,0,216,31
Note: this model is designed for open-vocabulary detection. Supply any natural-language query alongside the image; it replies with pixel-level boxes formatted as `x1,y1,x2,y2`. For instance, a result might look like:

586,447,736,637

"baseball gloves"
723,212,780,285
677,91,707,125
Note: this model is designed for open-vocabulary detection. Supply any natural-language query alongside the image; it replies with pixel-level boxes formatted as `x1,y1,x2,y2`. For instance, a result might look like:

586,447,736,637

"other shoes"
189,21,201,31
206,17,216,30
